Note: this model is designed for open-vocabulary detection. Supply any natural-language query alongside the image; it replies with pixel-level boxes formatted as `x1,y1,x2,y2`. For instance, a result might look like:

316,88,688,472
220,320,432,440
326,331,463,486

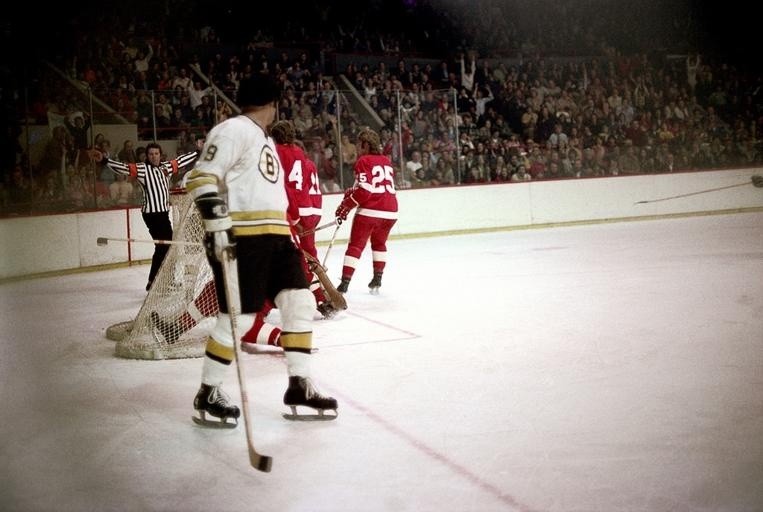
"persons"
295,139,320,284
84,138,205,298
150,180,312,353
270,120,335,319
334,128,400,295
183,72,338,422
0,1,763,219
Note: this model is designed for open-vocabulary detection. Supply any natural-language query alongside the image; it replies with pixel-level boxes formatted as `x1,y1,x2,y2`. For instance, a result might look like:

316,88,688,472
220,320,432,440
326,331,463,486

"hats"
236,70,284,109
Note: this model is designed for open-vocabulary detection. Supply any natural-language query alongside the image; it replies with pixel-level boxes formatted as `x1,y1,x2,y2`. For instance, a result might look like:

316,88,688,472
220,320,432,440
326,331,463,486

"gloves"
336,204,349,220
202,230,236,264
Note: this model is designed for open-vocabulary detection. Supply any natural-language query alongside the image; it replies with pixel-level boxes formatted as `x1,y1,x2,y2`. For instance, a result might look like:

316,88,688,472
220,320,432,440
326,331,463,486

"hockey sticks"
636,183,751,205
98,236,203,245
220,251,272,473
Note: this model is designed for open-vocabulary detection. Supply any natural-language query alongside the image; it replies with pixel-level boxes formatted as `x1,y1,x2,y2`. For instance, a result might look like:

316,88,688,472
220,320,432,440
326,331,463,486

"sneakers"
283,376,337,409
368,278,380,288
152,312,180,345
193,384,240,418
337,282,347,292
